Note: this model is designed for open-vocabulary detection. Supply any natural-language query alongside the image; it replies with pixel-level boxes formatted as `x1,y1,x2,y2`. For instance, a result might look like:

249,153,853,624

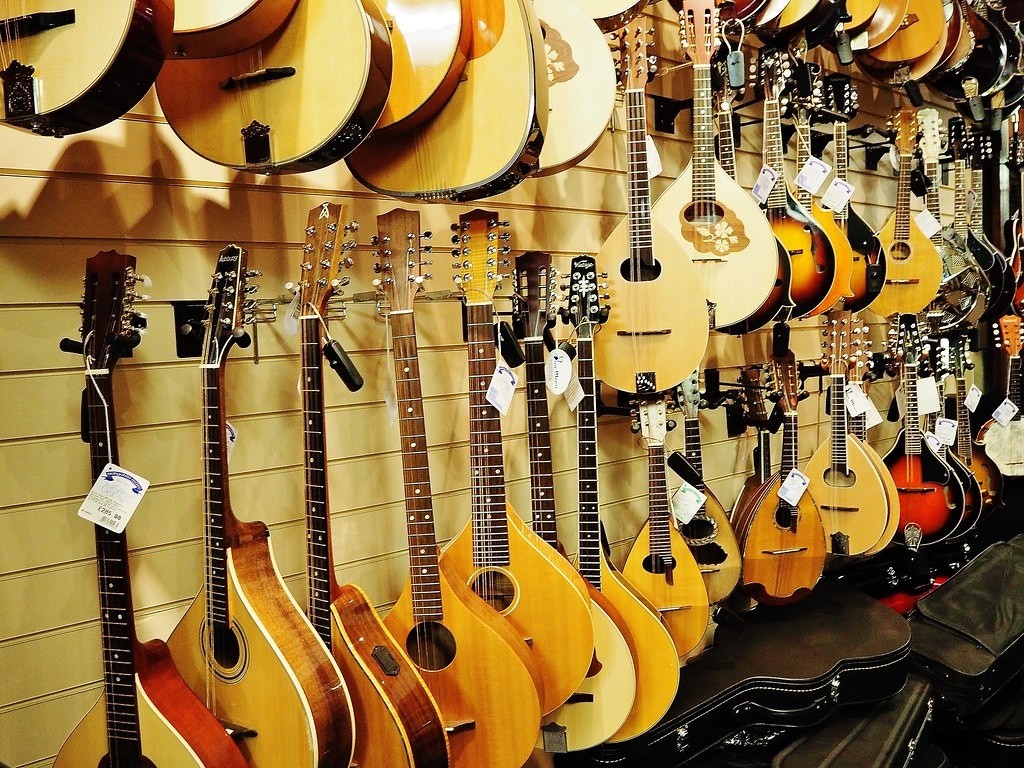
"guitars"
440,211,595,719
166,241,357,768
52,248,250,768
511,250,640,753
0,0,1024,609
371,208,547,768
621,398,711,658
651,0,780,330
559,256,680,745
594,12,710,394
286,200,453,768
